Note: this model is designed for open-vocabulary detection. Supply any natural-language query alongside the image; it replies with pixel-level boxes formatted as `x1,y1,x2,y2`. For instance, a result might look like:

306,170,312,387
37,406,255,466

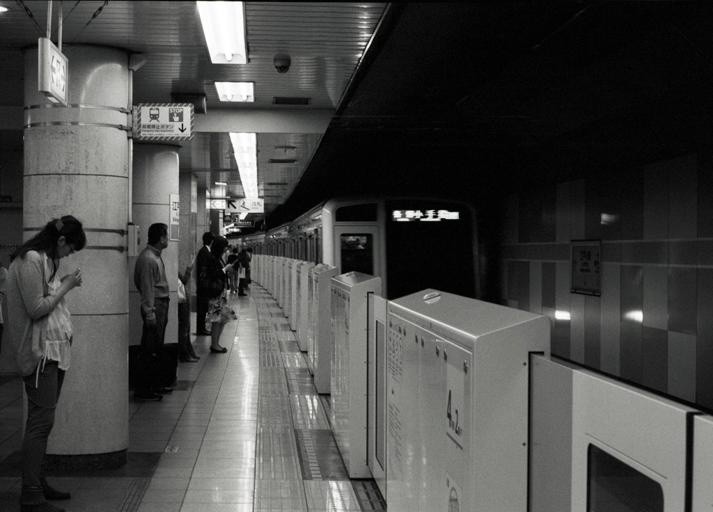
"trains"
226,196,482,301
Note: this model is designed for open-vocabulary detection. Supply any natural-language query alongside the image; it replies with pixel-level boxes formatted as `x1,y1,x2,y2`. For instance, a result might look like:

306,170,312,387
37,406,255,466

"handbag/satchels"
132,338,168,375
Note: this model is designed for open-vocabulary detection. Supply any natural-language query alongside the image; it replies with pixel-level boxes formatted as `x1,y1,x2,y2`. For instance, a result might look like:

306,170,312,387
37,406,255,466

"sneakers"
20,479,70,511
238,292,247,296
133,387,173,402
180,350,201,363
197,331,211,336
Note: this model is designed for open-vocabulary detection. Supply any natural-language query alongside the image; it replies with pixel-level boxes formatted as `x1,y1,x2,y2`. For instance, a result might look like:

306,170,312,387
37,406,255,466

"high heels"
210,345,226,353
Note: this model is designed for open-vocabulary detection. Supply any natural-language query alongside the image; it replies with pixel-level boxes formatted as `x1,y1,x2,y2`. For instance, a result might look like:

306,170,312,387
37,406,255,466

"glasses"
67,243,74,254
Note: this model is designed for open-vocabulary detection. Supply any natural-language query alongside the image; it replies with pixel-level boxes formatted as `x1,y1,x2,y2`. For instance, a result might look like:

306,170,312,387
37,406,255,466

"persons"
222,244,253,297
131,222,174,402
195,231,214,336
205,235,237,354
177,263,200,362
3,215,86,511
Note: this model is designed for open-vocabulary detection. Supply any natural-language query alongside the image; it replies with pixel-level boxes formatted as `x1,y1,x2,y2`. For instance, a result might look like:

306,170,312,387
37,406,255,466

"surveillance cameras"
273,55,291,73
